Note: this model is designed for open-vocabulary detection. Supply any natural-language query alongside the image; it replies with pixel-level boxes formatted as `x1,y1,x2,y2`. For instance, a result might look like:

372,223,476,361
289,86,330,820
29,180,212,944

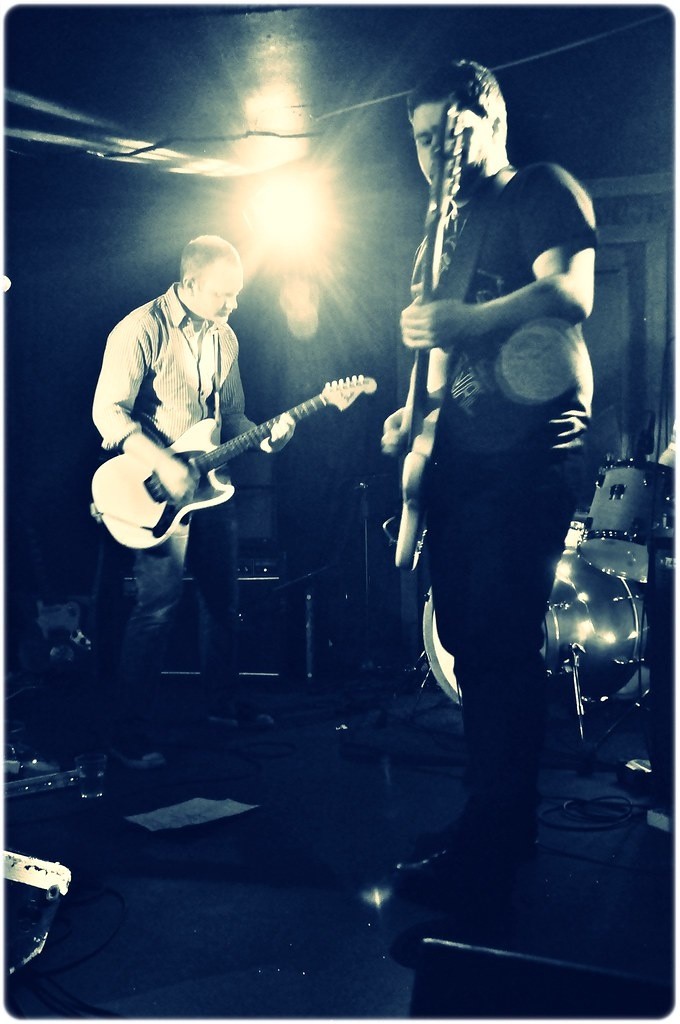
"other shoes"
208,714,275,729
109,749,166,771
418,814,539,862
391,838,515,901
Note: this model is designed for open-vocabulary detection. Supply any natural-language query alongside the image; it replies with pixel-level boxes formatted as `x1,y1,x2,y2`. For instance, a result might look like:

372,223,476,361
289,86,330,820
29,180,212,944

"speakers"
409,939,674,1018
122,574,286,678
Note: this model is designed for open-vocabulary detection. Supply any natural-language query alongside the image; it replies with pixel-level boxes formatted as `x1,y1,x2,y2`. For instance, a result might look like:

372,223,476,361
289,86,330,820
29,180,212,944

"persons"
92,237,295,771
382,61,598,891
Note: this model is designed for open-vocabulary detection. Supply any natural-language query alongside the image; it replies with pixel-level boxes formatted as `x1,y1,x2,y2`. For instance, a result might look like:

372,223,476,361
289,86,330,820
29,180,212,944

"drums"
577,459,675,584
420,543,648,708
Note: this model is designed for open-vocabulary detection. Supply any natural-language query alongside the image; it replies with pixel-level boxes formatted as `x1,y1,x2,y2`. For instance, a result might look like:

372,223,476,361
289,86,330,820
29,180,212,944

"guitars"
394,96,461,574
89,373,377,549
